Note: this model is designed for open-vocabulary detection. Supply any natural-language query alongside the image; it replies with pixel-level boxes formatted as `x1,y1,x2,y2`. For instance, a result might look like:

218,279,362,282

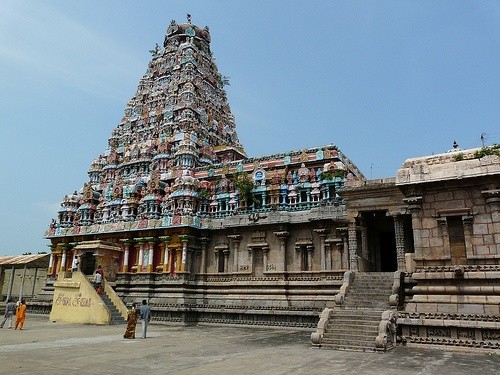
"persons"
0,299,16,329
72,256,79,272
98,265,104,280
93,271,102,291
123,303,137,339
139,300,151,339
15,300,26,330
97,286,102,296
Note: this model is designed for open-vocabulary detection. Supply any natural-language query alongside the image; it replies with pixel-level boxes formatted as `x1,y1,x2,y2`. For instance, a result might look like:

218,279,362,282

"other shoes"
0,326,3,328
